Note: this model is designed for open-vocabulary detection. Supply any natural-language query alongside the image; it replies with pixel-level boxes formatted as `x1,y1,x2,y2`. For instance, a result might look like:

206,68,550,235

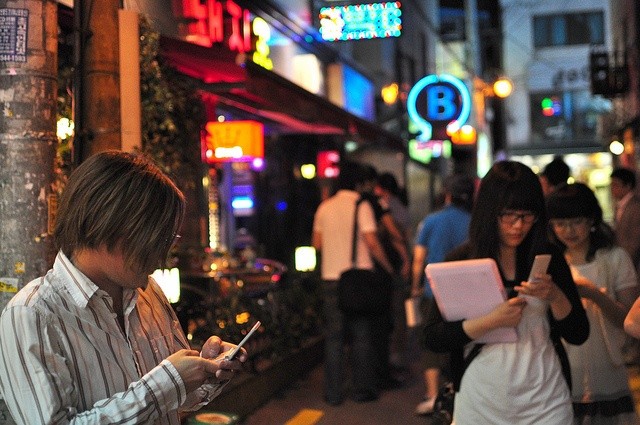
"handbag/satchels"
338,268,392,315
430,382,454,425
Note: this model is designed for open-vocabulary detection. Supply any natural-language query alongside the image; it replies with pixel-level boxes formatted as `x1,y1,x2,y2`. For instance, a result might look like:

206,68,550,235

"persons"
539,154,570,199
411,171,476,415
374,173,410,334
623,297,640,339
309,161,393,404
358,165,411,391
1,151,247,425
547,183,638,424
422,160,590,425
610,169,640,364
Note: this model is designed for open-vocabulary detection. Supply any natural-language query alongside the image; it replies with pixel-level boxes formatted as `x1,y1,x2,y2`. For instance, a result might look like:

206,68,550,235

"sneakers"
416,394,437,415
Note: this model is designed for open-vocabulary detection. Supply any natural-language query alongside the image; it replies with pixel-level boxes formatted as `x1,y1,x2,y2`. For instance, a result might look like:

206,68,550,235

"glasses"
498,209,541,224
167,234,181,247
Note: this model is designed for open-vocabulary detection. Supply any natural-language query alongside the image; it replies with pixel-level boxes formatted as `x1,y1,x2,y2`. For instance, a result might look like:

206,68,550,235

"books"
424,258,519,344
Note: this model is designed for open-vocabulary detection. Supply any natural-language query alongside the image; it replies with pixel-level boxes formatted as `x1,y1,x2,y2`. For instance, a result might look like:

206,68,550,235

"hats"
445,172,475,195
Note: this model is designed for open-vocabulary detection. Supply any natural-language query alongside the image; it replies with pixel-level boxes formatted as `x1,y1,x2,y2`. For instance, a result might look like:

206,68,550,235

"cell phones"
527,254,552,285
207,319,262,367
